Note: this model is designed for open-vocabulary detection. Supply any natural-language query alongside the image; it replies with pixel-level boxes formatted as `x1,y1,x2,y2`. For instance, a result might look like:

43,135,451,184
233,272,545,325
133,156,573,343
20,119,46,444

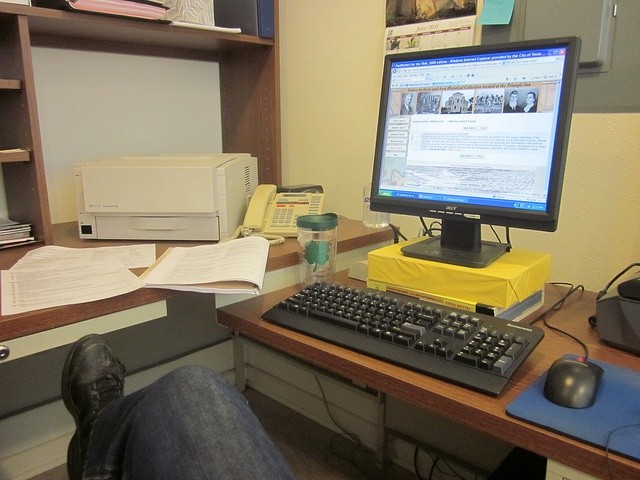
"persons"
523,91,536,112
400,92,414,115
503,90,525,113
61,333,295,480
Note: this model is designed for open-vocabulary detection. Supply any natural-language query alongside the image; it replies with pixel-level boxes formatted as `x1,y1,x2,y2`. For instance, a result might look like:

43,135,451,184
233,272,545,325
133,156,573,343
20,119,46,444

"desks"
217,249,640,479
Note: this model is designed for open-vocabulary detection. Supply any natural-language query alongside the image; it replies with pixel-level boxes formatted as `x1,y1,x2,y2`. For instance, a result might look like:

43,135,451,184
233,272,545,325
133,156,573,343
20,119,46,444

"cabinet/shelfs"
0,1,399,479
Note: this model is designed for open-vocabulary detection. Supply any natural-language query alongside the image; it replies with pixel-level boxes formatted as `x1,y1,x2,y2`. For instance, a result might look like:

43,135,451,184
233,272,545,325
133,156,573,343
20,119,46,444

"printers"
72,152,259,242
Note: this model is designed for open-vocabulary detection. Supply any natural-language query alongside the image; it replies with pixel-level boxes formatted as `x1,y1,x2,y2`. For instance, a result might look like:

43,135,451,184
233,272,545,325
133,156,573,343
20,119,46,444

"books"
1,216,43,250
137,235,270,296
363,276,544,323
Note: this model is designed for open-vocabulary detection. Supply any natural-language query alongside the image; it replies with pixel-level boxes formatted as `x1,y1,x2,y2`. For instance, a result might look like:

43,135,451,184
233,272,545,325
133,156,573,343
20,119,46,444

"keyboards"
259,280,546,396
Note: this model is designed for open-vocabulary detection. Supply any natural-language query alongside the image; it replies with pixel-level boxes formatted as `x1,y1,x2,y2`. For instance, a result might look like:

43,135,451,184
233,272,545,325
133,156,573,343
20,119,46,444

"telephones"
217,183,325,246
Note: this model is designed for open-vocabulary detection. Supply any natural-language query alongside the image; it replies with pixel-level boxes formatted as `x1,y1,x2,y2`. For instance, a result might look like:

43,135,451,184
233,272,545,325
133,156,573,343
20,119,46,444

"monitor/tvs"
370,34,583,268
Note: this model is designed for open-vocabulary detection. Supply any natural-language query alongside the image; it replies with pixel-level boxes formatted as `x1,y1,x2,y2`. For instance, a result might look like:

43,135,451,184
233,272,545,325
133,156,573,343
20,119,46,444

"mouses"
546,352,605,410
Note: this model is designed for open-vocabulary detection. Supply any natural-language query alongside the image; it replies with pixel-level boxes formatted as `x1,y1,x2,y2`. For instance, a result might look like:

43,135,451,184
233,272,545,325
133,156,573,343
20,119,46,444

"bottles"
297,214,337,291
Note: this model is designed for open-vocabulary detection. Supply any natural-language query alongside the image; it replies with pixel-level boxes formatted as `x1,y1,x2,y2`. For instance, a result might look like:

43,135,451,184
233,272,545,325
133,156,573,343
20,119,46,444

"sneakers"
61,334,125,480
